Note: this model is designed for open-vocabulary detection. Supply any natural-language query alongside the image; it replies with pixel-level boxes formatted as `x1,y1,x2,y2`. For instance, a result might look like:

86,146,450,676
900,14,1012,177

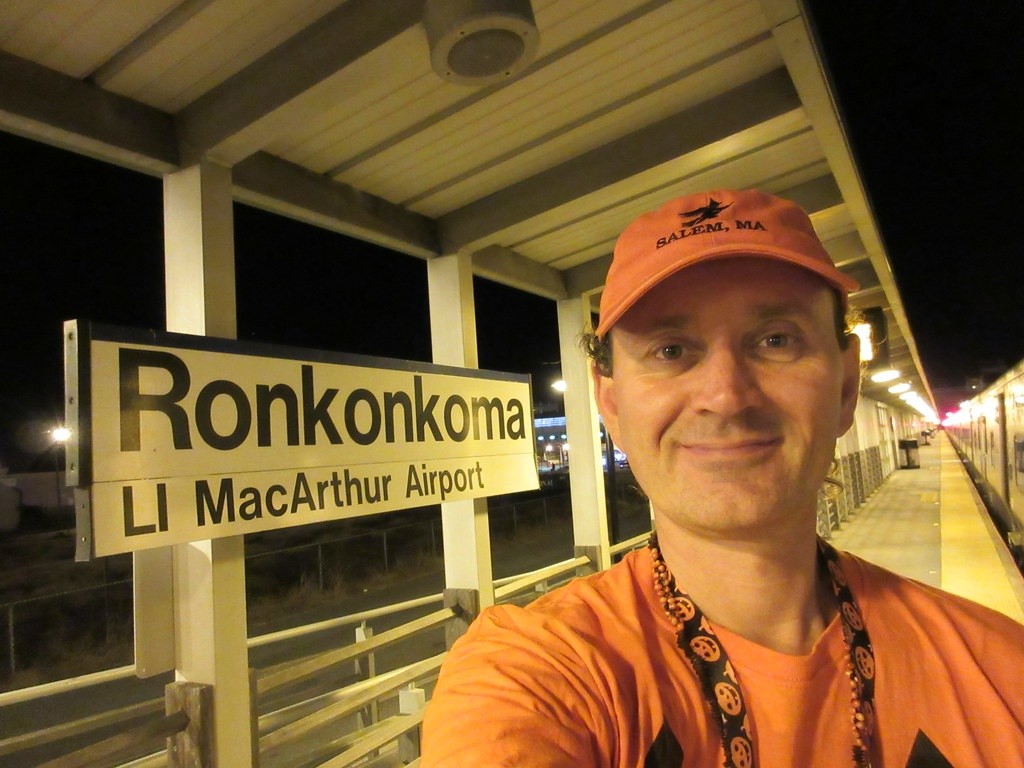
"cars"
543,467,569,489
618,460,629,467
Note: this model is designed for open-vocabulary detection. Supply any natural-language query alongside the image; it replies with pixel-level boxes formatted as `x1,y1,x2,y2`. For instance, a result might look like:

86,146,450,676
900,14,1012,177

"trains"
942,358,1024,529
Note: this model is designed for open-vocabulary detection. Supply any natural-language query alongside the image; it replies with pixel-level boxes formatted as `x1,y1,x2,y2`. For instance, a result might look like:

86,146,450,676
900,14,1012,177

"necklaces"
646,528,872,768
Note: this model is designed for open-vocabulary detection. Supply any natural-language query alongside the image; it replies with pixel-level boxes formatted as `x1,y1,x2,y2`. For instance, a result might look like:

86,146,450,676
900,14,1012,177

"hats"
595,188,860,342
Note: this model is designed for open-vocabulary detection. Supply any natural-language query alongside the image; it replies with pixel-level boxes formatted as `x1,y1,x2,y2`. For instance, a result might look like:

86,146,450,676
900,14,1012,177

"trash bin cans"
898,438,920,469
920,430,930,445
928,428,934,438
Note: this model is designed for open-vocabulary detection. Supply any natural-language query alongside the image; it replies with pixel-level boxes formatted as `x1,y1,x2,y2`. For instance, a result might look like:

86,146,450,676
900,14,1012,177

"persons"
421,190,1024,767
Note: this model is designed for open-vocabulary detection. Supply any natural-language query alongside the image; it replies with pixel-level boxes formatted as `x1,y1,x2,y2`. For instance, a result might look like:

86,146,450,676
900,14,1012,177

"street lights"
48,424,67,536
